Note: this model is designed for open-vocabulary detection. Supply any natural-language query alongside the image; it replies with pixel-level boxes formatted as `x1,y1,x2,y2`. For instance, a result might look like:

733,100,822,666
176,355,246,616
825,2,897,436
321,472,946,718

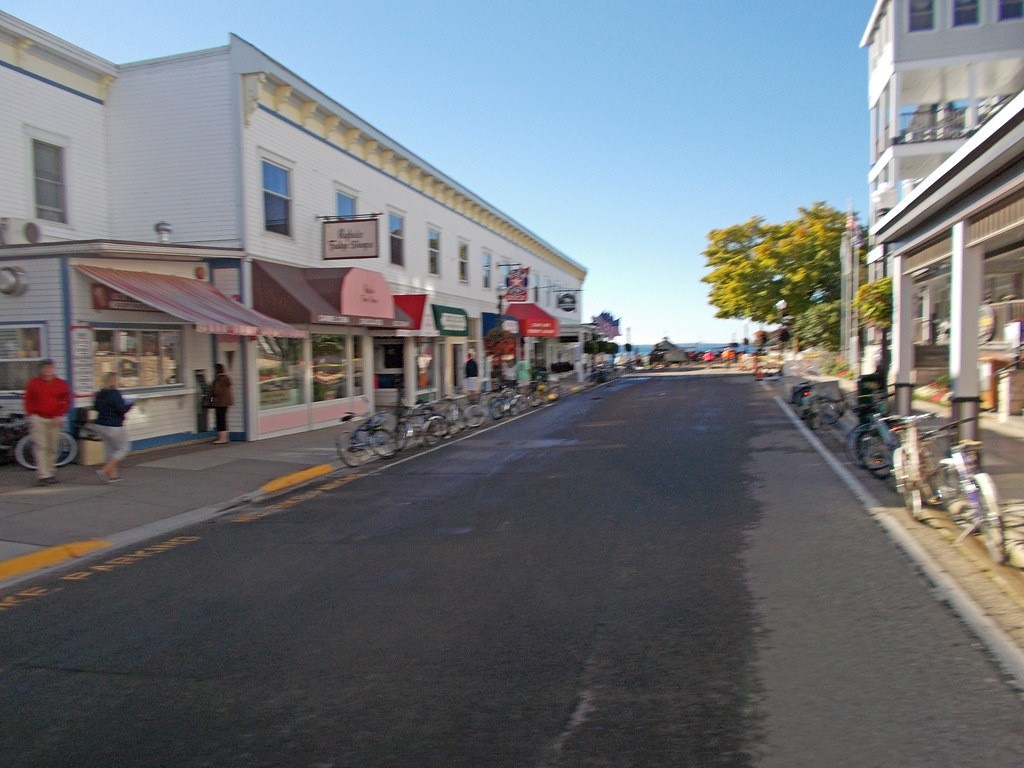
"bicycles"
526,381,563,408
843,384,899,480
877,413,940,520
792,381,844,431
334,412,400,468
488,382,532,421
918,415,1007,564
0,412,80,474
443,397,490,435
390,398,449,452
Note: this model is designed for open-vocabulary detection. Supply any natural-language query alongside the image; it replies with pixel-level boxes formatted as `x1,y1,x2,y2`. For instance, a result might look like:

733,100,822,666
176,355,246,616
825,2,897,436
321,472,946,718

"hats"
37,358,55,367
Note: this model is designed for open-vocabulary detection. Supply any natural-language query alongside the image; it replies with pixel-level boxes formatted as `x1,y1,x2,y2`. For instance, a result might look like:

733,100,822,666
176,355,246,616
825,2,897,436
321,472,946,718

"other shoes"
47,478,56,484
39,479,47,486
95,469,108,484
110,477,123,482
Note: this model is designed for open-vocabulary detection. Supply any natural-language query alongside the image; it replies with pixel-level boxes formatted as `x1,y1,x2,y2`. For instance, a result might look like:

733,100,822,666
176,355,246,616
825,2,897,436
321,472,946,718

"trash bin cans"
72,407,107,466
858,374,887,424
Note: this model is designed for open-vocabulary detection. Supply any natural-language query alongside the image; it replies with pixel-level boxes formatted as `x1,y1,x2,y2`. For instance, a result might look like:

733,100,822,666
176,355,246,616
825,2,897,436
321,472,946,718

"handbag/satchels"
228,393,234,405
203,393,215,408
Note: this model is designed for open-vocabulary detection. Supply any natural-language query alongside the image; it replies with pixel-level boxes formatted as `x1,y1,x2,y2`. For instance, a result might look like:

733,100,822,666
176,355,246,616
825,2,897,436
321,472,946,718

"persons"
206,362,236,445
94,372,135,485
24,357,72,484
464,350,532,402
702,347,735,369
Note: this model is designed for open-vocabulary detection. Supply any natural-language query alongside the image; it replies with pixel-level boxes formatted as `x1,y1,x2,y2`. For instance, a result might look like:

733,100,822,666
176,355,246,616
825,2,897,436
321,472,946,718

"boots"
211,431,229,444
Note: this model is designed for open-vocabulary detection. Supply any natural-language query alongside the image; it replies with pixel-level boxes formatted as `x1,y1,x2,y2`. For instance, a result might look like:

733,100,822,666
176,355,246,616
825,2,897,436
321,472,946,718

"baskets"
528,381,539,389
468,393,480,404
930,432,952,457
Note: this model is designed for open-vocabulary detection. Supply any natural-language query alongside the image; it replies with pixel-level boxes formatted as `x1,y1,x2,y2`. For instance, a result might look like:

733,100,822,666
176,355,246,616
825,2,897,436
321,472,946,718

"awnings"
74,266,309,340
